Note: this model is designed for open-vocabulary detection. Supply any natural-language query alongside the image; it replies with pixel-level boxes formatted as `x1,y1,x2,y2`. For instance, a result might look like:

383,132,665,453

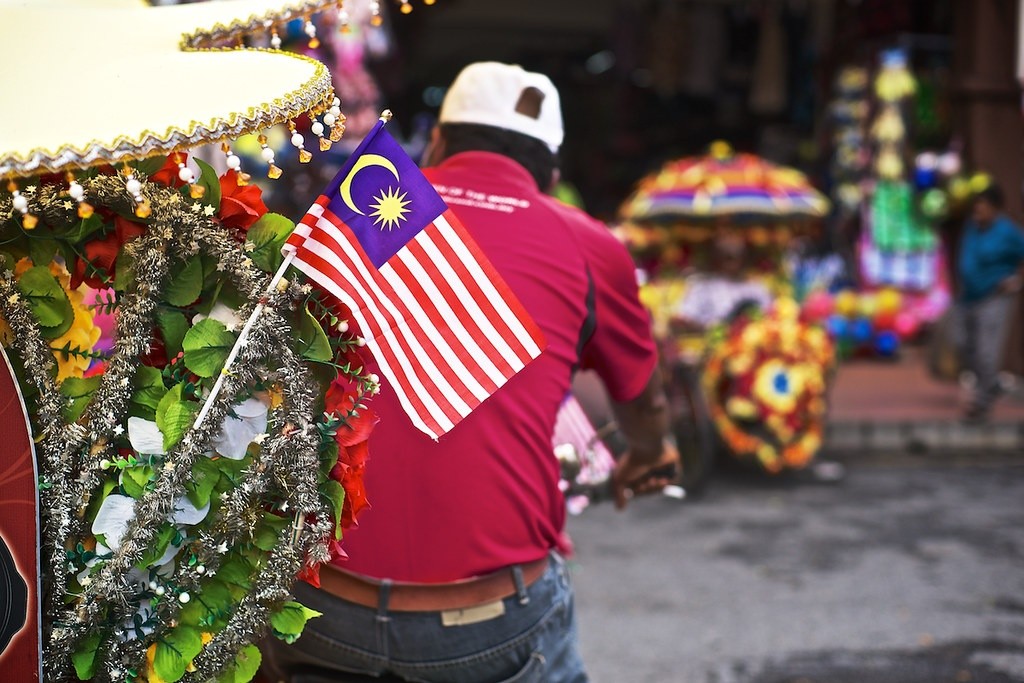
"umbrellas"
615,135,829,257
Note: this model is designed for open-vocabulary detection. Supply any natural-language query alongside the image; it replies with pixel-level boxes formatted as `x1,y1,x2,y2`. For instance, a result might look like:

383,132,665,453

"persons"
957,182,1024,420
257,62,679,683
632,243,681,327
671,231,773,325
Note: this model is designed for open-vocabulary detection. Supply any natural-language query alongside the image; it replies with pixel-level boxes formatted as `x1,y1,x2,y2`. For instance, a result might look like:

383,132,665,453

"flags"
280,118,545,444
545,396,615,514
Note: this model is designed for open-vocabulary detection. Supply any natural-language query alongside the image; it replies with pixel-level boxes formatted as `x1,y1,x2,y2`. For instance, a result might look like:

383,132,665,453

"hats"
438,60,565,154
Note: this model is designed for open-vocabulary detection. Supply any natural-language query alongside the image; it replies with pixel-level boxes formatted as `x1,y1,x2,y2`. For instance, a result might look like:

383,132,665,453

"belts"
297,554,551,612
